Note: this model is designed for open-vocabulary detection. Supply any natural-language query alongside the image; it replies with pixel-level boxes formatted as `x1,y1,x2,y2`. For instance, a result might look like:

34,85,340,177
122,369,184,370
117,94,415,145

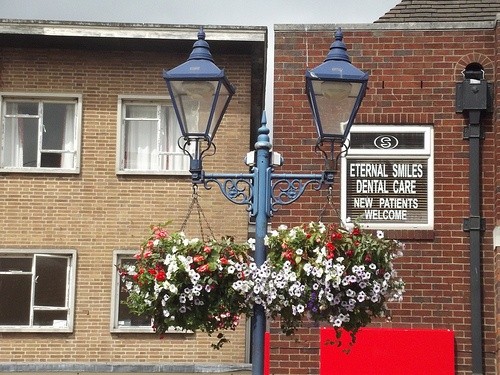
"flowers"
113,197,257,351
252,196,406,355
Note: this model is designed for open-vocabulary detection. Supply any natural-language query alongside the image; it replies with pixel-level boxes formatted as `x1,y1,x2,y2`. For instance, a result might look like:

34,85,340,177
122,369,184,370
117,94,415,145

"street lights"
114,27,406,375
